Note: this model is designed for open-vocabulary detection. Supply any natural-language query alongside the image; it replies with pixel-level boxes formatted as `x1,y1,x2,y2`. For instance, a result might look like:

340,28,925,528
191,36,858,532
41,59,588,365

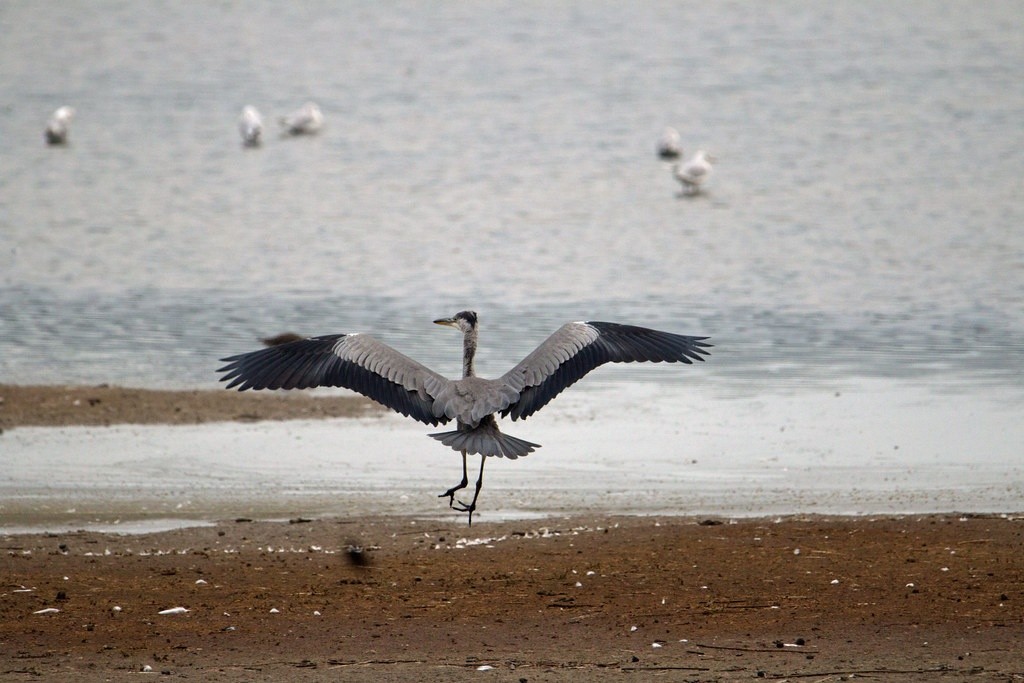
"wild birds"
657,125,714,198
238,102,264,149
281,101,325,138
215,310,715,527
43,106,77,145
